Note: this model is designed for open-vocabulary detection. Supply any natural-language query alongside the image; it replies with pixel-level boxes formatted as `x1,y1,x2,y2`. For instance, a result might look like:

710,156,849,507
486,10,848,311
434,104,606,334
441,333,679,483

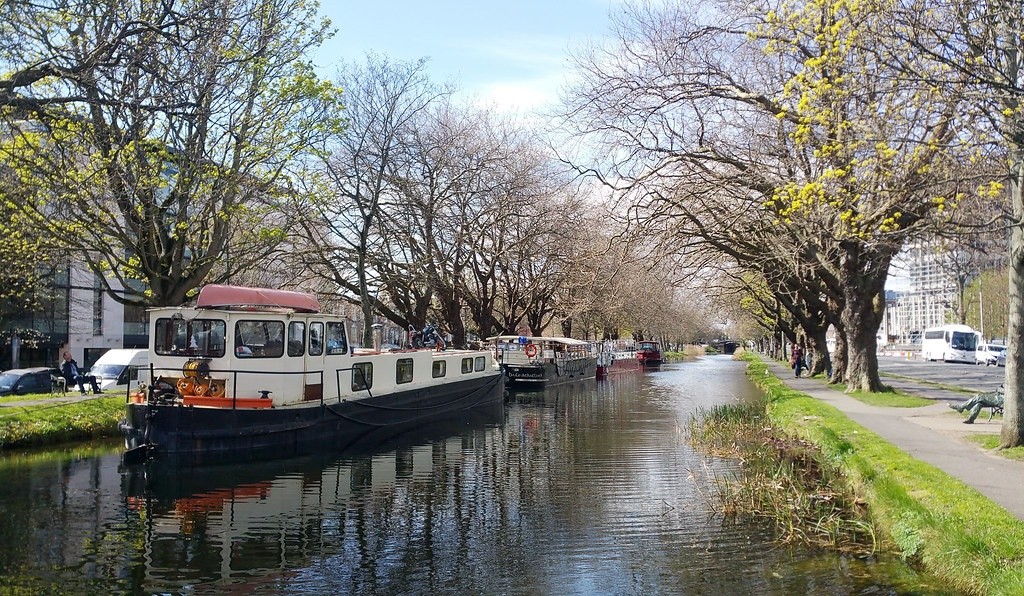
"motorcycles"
407,316,447,351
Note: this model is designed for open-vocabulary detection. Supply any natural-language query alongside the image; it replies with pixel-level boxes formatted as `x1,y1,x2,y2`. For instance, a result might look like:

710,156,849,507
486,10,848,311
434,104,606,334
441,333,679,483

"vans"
75,348,151,394
974,344,1007,367
0,368,68,397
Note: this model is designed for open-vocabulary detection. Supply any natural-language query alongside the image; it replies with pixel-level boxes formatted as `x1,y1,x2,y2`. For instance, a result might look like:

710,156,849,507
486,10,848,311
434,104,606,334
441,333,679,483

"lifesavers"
236,345,254,356
525,344,537,357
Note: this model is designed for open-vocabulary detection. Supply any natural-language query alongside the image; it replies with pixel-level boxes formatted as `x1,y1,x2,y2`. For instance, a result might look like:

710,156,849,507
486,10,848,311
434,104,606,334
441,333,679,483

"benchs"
47,366,102,398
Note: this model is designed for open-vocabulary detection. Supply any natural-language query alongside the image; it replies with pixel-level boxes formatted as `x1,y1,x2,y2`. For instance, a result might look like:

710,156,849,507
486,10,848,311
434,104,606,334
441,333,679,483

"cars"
997,350,1008,368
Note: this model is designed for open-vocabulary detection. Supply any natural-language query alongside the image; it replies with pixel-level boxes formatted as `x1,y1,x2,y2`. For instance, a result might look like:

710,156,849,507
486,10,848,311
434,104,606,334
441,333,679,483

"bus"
921,325,982,364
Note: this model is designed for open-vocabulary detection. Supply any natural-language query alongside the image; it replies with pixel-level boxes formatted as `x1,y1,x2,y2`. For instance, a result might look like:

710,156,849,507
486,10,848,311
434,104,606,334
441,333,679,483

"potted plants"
131,384,147,403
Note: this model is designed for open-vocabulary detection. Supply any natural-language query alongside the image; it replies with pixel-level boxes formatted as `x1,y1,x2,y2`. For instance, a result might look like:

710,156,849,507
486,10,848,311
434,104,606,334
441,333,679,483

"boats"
117,283,506,474
637,341,663,370
596,340,641,376
485,335,600,392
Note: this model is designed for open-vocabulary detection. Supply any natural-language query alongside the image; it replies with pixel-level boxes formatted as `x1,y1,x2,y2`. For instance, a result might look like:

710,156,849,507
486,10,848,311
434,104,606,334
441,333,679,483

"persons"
947,385,1004,424
791,343,803,379
60,351,104,395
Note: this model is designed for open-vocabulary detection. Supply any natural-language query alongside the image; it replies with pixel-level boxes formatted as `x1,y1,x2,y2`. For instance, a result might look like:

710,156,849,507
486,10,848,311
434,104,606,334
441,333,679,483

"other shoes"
963,419,974,424
94,390,104,394
81,393,87,396
947,402,964,413
